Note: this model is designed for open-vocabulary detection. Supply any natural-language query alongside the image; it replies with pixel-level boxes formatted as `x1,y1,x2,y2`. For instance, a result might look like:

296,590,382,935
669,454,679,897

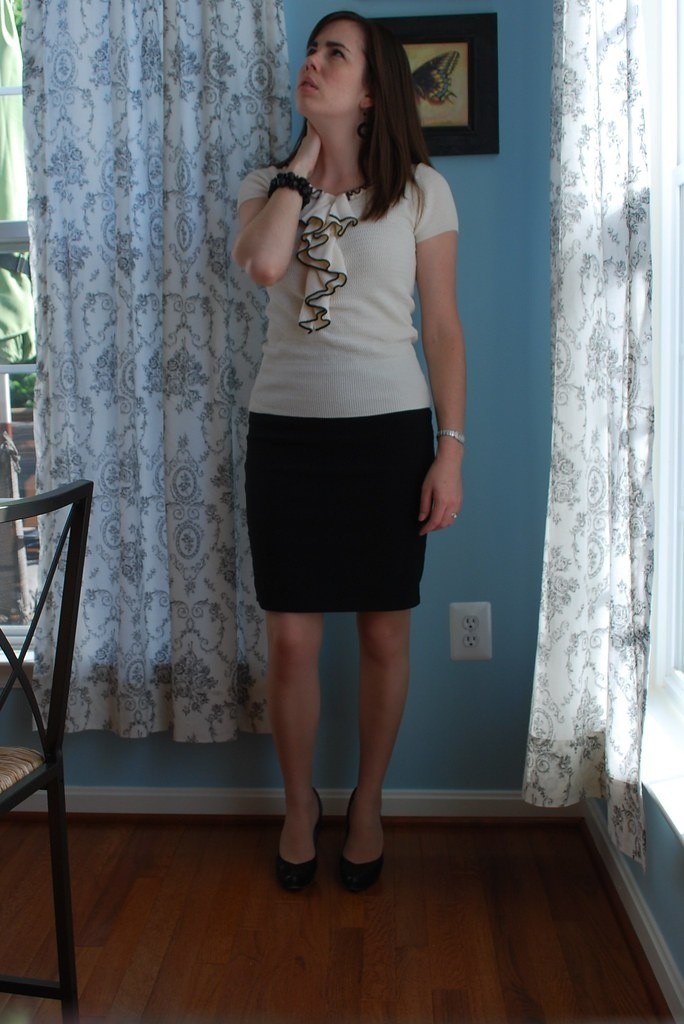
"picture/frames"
369,12,500,157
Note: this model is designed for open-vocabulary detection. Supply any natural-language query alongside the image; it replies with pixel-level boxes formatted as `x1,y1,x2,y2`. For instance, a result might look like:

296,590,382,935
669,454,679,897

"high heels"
337,786,386,891
274,788,324,895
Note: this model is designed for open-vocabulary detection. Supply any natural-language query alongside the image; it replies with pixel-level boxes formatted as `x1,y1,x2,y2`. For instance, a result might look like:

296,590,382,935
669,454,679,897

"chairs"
0,480,94,1024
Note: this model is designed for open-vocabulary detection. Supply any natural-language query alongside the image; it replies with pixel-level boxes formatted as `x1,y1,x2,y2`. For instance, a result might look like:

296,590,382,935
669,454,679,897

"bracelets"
436,430,464,445
269,172,311,209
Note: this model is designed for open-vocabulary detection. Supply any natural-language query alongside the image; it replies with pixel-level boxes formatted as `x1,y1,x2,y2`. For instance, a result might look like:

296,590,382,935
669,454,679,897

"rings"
451,513,457,519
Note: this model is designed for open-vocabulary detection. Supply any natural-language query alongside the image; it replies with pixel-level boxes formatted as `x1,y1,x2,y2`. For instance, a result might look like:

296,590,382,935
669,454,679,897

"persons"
234,10,466,892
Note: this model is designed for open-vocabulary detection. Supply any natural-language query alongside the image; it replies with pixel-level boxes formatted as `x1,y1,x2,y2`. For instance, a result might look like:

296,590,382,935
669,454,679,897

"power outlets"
449,601,492,662
